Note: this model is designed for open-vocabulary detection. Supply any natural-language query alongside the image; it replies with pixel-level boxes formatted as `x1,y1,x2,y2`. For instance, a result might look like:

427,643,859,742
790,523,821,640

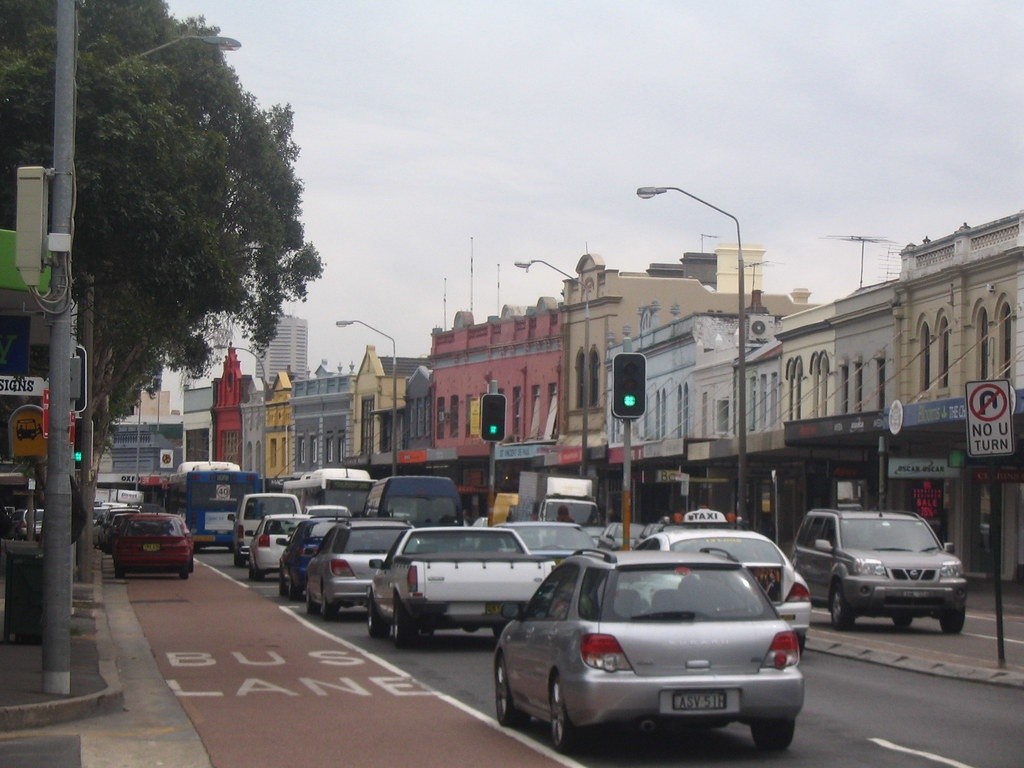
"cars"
1,503,193,580
638,507,812,655
495,521,600,556
595,520,701,549
243,505,417,617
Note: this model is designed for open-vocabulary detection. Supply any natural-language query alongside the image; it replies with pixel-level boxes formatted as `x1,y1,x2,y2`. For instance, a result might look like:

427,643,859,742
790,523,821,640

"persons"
556,506,576,523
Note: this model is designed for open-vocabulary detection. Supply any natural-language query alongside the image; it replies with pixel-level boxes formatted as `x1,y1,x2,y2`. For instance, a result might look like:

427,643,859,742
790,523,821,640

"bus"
281,470,376,519
164,461,262,551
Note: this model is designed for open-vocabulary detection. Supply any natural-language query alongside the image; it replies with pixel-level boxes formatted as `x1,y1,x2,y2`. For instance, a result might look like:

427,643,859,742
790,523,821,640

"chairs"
613,587,679,619
415,543,439,554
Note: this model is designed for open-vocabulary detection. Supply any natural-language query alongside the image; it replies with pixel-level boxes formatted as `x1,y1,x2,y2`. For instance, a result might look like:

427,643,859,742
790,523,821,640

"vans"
492,493,522,525
229,491,302,566
361,477,464,531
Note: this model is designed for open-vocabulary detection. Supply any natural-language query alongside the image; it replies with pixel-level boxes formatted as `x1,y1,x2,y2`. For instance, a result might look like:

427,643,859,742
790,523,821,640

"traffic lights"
481,393,506,442
613,353,648,417
73,420,95,473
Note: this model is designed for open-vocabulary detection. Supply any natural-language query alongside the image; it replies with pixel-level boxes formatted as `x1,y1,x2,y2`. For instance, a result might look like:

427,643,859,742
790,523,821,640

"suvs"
494,546,805,753
791,506,968,634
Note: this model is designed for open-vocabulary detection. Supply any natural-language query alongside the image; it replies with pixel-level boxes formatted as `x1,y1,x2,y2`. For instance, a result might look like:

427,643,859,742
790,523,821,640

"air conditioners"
747,315,776,342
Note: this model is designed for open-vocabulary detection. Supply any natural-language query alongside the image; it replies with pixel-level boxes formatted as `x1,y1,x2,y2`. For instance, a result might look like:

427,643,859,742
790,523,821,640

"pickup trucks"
363,531,555,648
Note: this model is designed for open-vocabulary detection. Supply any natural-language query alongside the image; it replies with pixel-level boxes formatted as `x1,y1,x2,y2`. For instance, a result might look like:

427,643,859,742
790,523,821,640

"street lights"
212,320,397,475
638,187,751,530
514,260,589,476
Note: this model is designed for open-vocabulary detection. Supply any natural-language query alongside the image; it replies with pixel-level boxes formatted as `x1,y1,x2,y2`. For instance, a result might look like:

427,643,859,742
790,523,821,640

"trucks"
516,470,606,542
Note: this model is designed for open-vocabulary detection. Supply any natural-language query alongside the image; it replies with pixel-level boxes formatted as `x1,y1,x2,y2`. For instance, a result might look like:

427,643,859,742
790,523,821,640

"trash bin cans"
4,546,46,635
